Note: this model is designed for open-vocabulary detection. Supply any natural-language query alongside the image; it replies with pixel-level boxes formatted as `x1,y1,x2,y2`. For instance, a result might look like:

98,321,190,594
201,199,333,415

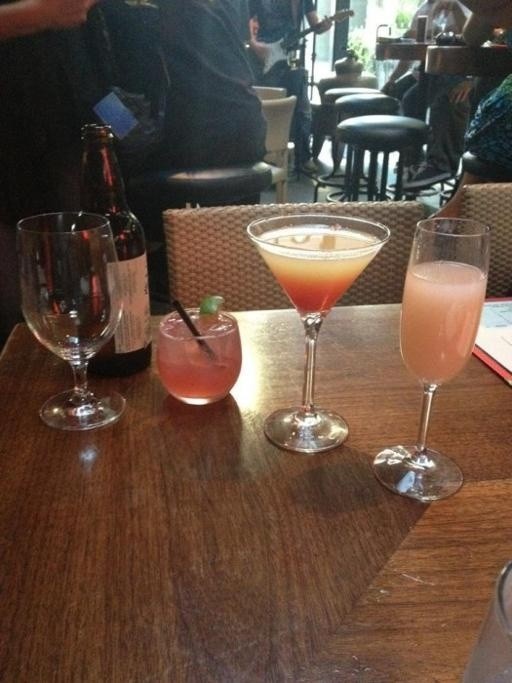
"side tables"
375,37,512,75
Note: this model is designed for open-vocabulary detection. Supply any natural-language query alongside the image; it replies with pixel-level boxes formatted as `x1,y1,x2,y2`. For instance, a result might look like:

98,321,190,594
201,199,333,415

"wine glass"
247,212,391,454
14,211,127,432
371,216,491,501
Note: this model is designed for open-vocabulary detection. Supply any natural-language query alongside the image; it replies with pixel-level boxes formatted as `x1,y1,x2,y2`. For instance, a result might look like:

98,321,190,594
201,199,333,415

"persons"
407,0,487,188
0,0,98,39
379,0,467,174
96,0,266,301
246,0,332,175
432,0,512,217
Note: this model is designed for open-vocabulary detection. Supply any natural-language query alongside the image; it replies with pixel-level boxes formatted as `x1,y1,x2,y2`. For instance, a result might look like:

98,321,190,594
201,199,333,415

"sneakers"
296,160,319,174
401,162,455,191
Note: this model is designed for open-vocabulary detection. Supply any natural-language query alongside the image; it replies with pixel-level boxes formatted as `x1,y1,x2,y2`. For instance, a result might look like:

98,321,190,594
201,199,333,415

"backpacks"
91,0,170,157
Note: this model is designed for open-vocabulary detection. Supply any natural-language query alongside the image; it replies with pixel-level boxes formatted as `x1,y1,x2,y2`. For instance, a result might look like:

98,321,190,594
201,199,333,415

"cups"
431,17,446,41
461,563,512,683
158,308,242,406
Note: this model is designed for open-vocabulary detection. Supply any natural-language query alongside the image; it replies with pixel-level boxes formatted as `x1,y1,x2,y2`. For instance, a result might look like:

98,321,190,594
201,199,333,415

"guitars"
242,9,353,77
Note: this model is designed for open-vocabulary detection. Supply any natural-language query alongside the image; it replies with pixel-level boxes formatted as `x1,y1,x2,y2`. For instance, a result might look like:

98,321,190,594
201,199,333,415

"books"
471,298,512,387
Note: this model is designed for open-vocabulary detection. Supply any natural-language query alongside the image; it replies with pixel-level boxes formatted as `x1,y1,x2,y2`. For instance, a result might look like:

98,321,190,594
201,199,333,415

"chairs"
163,200,426,311
262,94,297,202
254,86,286,99
458,183,511,297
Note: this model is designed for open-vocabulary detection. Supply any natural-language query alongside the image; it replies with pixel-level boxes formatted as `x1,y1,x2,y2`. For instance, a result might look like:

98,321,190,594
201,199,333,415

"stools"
168,159,273,205
336,114,426,202
326,94,400,201
317,86,381,187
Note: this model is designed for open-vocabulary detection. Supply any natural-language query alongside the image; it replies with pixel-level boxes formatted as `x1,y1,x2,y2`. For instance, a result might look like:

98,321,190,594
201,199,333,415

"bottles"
76,122,153,379
